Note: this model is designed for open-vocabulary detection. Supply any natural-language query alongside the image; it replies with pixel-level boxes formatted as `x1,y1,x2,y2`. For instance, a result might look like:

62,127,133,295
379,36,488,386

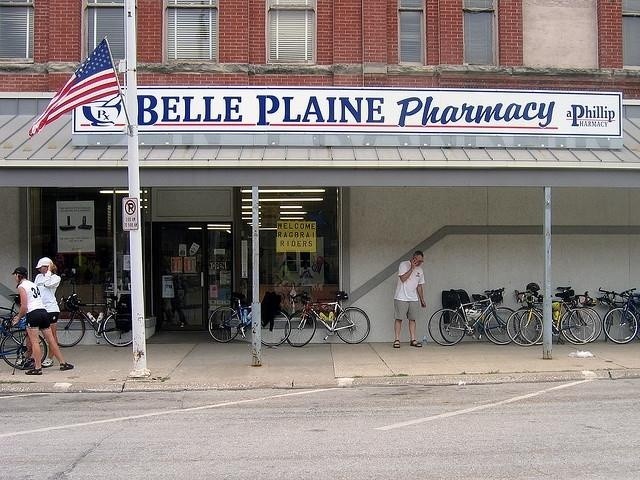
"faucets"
410,340,423,348
393,339,401,348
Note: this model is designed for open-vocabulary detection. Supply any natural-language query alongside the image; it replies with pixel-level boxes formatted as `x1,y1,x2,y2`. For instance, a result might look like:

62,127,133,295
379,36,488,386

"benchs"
29,39,119,139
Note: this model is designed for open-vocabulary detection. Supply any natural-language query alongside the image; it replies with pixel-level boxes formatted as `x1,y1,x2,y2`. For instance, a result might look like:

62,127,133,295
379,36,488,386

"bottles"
12,267,29,276
35,257,54,269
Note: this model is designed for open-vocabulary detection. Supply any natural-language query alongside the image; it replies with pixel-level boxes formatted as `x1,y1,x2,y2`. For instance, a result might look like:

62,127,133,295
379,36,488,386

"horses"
414,257,417,262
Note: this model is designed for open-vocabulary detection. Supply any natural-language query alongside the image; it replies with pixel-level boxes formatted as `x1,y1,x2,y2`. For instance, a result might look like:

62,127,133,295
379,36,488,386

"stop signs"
283,289,371,347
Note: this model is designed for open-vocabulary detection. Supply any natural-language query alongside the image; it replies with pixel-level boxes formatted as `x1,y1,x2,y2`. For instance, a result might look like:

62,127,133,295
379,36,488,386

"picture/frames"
259,283,339,314
94,285,104,313
56,284,92,311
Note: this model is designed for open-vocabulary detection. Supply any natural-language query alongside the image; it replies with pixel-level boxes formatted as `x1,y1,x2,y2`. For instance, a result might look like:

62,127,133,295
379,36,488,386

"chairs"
16,358,28,364
41,359,54,367
24,368,42,376
60,363,73,371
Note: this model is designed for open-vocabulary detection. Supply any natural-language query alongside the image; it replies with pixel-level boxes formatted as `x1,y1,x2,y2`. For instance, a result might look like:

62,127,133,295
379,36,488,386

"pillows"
466,307,481,318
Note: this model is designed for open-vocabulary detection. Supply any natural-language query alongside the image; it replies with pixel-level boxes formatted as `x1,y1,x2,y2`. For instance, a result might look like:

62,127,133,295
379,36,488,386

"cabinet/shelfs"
243,309,247,322
86,311,96,323
328,310,333,321
554,308,560,320
247,310,252,320
318,311,328,321
97,312,103,323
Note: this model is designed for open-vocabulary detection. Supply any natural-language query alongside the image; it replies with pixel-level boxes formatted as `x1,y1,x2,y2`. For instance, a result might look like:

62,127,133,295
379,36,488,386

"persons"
11,267,73,375
17,257,61,368
280,256,293,282
393,251,426,348
299,260,315,286
